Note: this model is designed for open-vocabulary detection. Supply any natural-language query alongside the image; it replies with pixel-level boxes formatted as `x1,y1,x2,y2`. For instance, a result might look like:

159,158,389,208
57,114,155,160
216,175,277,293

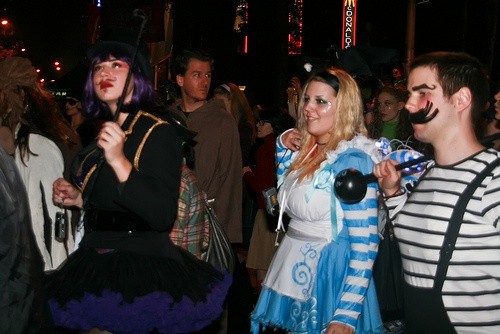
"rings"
296,137,303,141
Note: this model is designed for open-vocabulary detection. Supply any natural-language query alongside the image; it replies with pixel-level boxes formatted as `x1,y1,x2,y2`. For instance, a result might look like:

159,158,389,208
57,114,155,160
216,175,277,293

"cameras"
263,185,279,216
55,211,69,242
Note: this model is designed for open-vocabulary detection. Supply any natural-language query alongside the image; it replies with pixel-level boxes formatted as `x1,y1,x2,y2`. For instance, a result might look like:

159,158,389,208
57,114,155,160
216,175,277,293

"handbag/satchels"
201,205,236,276
373,219,404,324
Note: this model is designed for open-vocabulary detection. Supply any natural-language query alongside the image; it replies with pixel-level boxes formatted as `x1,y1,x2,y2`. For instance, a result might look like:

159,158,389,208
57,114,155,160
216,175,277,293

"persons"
0,41,500,334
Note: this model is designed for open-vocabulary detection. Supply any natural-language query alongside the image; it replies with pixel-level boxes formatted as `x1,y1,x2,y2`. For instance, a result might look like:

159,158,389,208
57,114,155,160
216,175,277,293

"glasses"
64,99,79,106
257,120,270,126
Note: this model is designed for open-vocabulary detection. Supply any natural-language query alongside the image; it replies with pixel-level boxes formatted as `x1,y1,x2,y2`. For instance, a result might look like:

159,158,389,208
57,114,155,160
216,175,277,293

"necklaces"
274,141,329,246
